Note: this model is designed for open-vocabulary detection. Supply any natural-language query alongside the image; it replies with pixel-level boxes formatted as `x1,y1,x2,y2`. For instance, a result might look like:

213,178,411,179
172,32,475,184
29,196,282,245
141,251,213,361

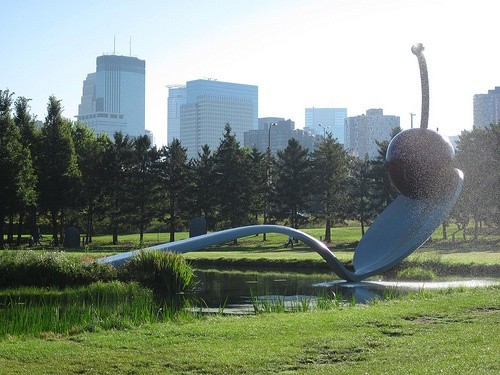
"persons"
283,226,294,249
31,228,43,246
